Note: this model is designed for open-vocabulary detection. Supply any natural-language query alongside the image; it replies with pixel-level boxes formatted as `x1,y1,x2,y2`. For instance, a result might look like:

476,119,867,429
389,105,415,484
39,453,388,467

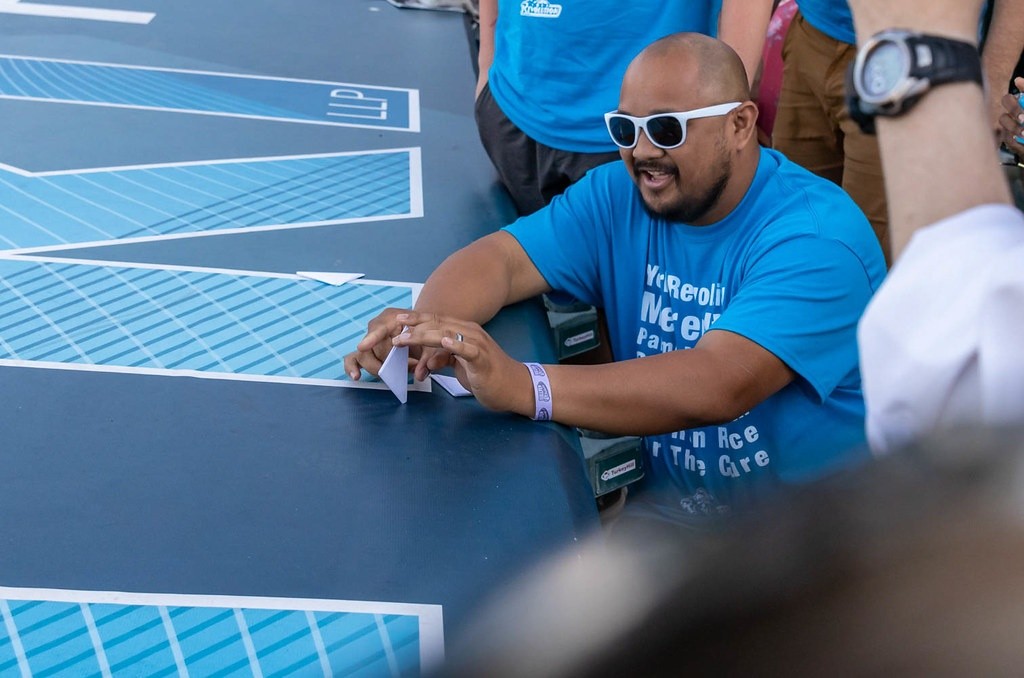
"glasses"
605,101,743,149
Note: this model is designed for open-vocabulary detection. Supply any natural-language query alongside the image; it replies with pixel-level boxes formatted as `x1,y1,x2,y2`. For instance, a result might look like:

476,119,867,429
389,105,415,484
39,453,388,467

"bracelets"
520,362,552,422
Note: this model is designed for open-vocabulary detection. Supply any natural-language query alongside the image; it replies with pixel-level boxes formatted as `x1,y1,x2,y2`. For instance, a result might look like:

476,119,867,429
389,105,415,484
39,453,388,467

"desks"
0,0,602,678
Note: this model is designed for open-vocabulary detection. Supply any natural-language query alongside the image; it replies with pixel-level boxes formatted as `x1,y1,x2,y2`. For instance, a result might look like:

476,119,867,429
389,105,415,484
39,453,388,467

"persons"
457,1,1024,678
346,30,878,678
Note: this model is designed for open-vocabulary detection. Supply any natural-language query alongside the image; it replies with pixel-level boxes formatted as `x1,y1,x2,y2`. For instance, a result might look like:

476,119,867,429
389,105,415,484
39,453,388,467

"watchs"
846,32,987,137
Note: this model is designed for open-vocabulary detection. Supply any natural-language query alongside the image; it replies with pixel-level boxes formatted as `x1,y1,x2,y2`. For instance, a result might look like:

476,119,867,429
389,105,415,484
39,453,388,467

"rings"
457,332,463,343
